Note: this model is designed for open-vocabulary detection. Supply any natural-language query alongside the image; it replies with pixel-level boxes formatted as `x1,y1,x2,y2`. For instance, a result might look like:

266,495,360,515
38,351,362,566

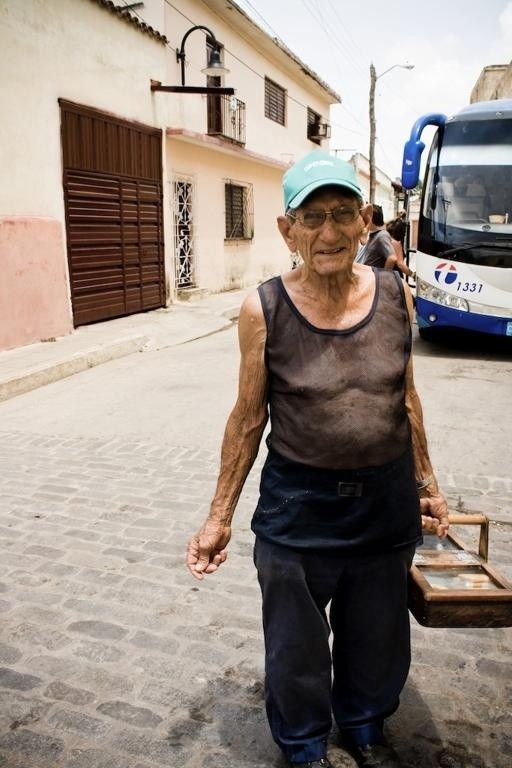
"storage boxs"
407,514,511,628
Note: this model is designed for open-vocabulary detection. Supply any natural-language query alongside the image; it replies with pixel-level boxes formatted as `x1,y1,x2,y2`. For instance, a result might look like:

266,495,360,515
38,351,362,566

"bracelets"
413,474,436,489
410,271,416,278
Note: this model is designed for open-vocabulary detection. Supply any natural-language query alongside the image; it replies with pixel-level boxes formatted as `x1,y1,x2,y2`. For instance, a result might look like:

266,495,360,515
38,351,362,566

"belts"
322,481,366,498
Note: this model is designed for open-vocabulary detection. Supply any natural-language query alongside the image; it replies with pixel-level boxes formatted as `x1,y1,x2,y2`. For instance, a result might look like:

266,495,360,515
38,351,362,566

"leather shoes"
338,734,398,767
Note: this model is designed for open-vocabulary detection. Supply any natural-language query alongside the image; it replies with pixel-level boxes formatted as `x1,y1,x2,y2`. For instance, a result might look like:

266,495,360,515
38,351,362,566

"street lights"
368,63,415,204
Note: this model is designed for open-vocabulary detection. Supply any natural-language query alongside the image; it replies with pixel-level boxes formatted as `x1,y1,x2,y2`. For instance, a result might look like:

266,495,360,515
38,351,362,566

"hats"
283,148,363,212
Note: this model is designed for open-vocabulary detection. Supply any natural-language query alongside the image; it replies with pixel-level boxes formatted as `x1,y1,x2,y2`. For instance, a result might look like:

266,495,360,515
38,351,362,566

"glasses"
285,206,363,223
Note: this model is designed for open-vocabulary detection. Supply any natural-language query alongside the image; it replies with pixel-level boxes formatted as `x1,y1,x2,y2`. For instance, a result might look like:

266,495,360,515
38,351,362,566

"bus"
400,98,511,342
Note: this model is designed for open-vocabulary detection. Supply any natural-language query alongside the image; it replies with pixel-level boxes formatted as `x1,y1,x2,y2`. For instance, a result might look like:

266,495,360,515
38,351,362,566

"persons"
359,203,398,272
188,150,450,767
384,208,417,309
